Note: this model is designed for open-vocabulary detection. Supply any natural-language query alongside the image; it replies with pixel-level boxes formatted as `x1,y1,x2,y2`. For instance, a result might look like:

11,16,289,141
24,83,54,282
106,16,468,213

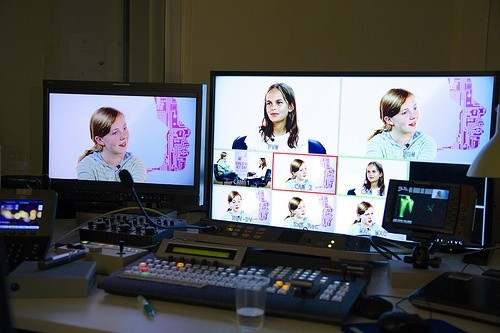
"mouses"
355,296,392,319
377,312,430,333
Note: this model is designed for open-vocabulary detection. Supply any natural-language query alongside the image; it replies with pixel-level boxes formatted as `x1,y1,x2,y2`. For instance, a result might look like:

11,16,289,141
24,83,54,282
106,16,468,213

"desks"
5,219,500,333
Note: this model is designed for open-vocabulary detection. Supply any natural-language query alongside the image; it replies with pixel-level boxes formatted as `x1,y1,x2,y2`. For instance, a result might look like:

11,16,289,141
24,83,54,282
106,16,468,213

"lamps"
466,104,499,179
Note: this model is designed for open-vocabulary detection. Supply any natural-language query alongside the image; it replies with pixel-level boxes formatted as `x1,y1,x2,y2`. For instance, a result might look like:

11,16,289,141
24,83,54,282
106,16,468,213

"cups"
234,275,270,333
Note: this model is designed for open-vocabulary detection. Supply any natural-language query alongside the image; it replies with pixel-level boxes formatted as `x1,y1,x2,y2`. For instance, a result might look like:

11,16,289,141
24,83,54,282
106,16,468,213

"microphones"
118,169,214,231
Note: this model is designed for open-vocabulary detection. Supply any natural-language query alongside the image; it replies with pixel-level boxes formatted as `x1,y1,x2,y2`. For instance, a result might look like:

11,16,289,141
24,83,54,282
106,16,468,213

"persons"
355,161,387,196
341,201,387,237
77,107,148,183
366,88,436,161
232,83,327,155
216,152,335,230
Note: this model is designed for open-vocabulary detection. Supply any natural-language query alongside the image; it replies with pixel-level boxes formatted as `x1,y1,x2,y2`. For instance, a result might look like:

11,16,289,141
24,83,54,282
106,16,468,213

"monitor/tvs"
40,77,207,204
382,180,474,241
0,188,59,238
204,69,499,252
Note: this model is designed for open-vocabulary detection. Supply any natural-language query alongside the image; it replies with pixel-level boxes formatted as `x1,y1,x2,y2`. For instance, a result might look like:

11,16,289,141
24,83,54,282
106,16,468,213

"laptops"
408,270,500,326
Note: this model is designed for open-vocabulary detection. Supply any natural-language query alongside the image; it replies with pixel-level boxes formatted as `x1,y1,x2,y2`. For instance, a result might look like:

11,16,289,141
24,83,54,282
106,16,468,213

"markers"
35,248,87,271
137,294,157,318
